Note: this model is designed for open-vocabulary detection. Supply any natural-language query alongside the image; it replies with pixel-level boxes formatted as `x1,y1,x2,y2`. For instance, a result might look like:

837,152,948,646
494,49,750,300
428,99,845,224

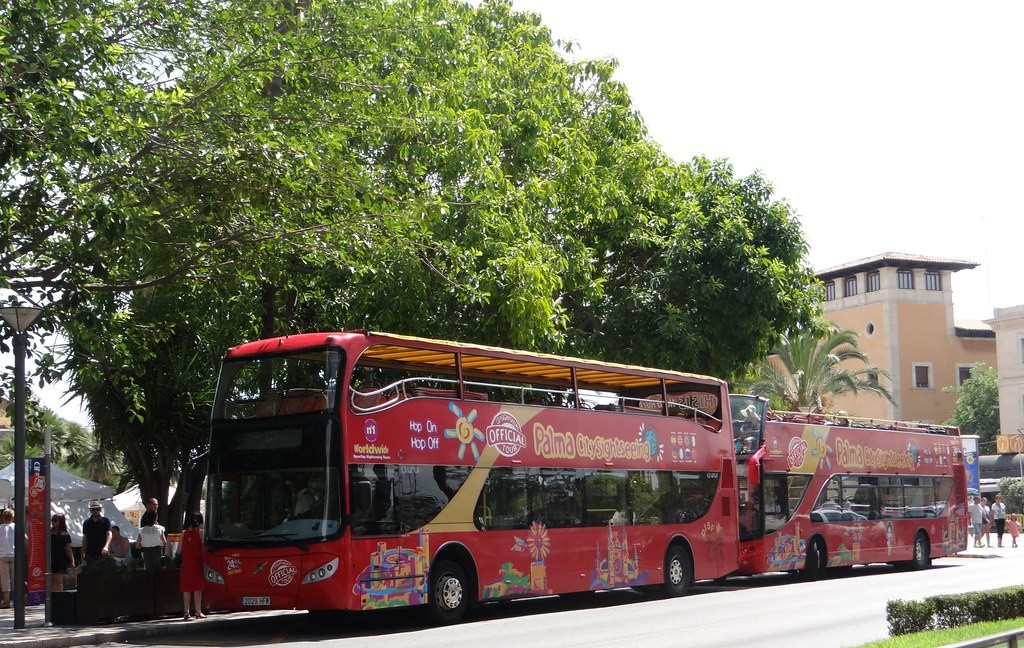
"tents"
105,484,205,529
0,459,113,502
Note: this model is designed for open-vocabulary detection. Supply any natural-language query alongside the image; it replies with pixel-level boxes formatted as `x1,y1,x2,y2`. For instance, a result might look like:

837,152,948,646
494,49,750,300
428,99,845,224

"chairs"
349,380,591,409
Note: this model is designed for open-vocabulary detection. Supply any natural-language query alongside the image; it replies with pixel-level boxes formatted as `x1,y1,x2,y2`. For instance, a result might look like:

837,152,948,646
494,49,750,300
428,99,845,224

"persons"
81,502,111,564
141,497,159,527
177,512,207,619
0,506,40,609
968,495,1021,548
108,526,132,558
51,515,75,591
740,404,783,452
135,511,167,557
837,411,849,427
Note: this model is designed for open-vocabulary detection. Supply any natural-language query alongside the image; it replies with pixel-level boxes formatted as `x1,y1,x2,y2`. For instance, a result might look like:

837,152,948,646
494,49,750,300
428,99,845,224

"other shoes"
0,602,10,608
987,546,992,547
998,546,1004,547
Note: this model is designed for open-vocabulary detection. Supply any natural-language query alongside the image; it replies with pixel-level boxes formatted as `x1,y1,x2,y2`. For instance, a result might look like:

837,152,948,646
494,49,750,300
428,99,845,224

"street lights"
0,305,47,628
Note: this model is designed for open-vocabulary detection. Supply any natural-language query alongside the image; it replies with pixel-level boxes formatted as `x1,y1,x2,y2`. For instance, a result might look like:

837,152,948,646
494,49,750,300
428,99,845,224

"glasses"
51,519,59,523
92,508,100,511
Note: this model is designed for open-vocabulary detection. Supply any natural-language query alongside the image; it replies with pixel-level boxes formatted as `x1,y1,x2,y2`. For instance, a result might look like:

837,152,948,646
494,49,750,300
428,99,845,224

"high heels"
196,611,207,619
184,611,191,621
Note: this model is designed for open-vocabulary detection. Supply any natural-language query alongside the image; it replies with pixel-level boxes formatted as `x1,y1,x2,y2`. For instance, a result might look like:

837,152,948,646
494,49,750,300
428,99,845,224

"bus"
200,329,742,627
729,393,970,583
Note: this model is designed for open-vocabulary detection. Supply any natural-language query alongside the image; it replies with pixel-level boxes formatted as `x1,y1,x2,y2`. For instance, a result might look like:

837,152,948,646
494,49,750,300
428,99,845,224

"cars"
810,498,951,524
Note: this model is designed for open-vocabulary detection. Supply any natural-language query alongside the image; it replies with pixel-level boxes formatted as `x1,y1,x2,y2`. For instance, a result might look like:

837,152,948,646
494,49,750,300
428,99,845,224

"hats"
89,501,102,509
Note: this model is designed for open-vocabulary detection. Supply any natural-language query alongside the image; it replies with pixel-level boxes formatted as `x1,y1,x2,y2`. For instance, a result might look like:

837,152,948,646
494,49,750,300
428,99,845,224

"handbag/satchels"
982,514,990,524
